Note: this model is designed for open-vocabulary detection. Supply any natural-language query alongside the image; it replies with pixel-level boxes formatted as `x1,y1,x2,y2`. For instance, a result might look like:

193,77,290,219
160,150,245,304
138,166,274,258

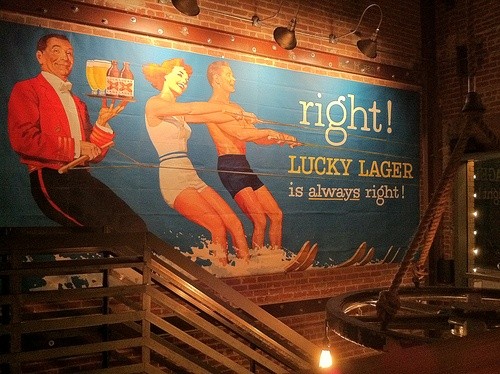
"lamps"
251,0,302,51
171,0,202,17
328,4,384,59
317,318,334,370
